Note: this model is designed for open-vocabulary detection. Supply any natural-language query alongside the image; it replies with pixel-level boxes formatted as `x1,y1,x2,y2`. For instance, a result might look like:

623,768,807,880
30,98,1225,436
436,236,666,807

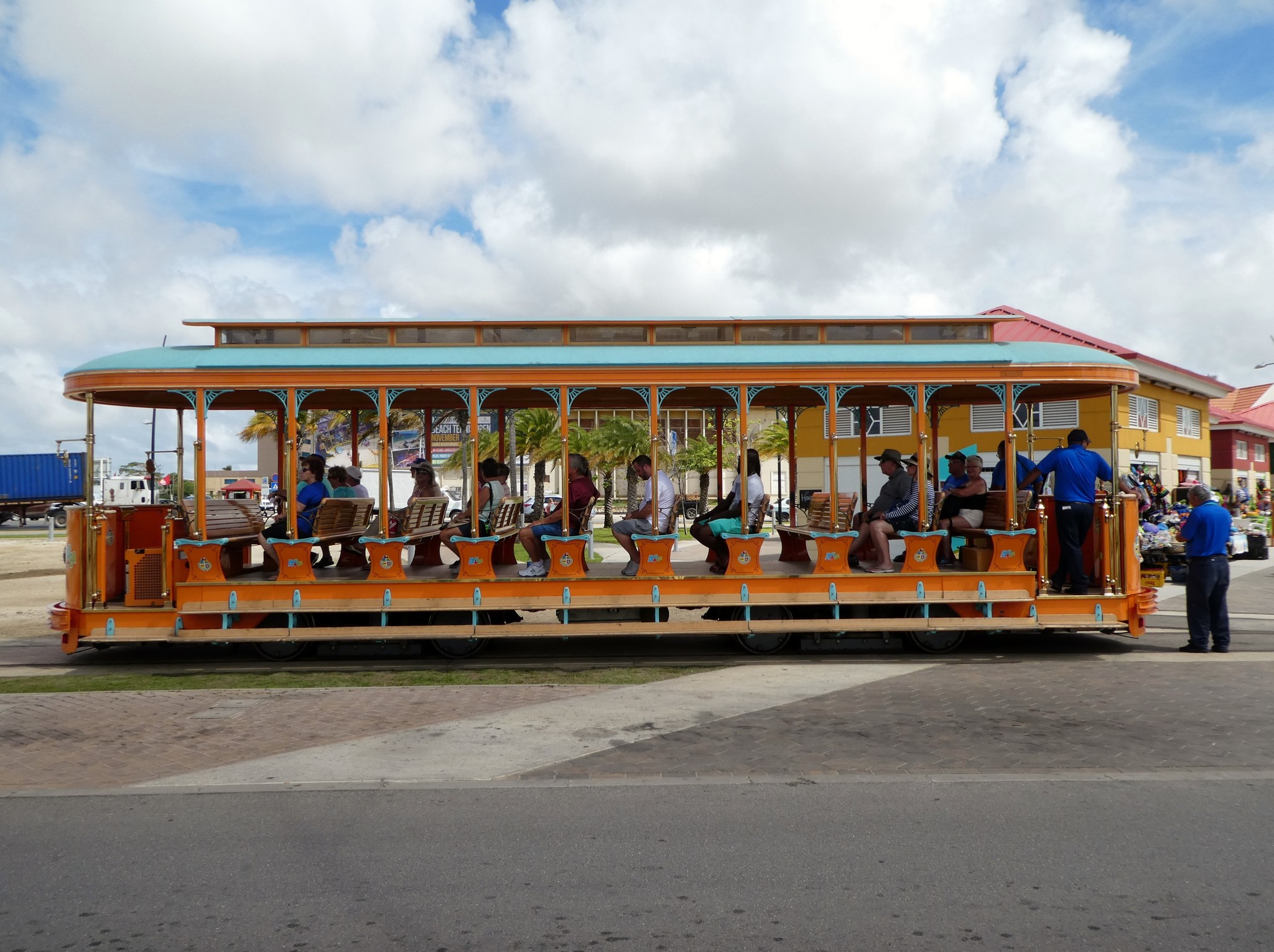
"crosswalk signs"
271,482,278,491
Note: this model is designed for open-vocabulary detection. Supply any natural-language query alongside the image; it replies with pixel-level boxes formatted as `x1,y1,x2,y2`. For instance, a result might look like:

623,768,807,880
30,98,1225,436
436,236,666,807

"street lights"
219,474,222,499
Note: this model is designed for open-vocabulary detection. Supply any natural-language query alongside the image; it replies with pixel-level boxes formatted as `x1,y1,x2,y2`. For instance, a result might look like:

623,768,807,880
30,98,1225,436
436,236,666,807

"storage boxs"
1226,516,1269,560
1140,560,1189,587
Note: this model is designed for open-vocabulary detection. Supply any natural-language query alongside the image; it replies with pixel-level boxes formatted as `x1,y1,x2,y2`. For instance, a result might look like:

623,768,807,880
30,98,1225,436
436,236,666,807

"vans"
185,496,213,499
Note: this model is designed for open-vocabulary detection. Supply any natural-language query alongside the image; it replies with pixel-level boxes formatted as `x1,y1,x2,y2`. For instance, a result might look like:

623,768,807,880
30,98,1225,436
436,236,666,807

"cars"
524,494,595,522
159,498,178,505
440,487,461,521
260,495,277,510
766,498,789,522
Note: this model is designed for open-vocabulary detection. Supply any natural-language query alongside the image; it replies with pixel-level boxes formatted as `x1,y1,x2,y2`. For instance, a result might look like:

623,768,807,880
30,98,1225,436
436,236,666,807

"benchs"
179,490,1037,584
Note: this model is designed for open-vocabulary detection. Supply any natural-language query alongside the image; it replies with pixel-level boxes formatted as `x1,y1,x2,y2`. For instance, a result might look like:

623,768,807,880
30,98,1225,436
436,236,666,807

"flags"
159,474,171,485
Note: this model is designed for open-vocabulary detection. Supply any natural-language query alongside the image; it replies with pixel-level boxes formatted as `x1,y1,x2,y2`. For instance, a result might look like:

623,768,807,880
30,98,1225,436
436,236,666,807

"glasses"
879,459,891,463
996,449,1002,458
327,474,337,479
417,471,428,476
964,466,981,470
907,462,915,467
303,467,311,472
737,455,740,460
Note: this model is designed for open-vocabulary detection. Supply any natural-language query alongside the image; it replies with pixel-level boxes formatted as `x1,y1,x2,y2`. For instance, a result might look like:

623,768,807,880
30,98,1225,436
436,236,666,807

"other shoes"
449,558,460,568
1051,582,1061,591
937,557,958,566
1065,585,1088,595
452,563,460,574
1179,643,1228,652
710,562,728,573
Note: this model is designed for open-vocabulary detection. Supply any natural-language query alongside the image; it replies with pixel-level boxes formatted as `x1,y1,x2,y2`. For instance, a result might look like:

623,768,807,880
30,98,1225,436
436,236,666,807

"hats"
345,466,362,480
874,449,904,469
406,458,427,468
900,452,934,478
1067,429,1092,443
945,451,967,464
299,453,325,466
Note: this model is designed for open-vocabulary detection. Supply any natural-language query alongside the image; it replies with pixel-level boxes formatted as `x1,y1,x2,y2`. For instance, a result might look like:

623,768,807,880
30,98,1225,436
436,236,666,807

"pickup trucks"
637,499,700,520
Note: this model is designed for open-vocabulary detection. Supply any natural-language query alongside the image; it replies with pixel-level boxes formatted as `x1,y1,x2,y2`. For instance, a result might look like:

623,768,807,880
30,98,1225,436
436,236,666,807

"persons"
935,455,987,566
611,455,675,576
690,448,765,574
342,458,442,569
440,457,511,573
315,434,337,468
893,451,970,563
518,453,601,577
989,440,1044,508
368,448,379,455
847,449,935,573
258,454,369,581
1017,429,1141,595
1175,486,1232,652
347,448,352,459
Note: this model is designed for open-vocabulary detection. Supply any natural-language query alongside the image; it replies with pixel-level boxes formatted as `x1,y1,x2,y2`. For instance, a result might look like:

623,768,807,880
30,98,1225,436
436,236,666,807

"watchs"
272,491,276,496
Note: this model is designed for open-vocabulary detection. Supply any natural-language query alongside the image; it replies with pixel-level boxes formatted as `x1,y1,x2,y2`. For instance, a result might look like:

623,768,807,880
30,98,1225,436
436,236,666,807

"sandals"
313,556,334,568
893,550,908,562
268,569,280,581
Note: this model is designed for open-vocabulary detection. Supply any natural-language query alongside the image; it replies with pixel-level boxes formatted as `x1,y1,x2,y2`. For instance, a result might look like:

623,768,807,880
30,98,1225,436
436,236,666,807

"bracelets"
371,450,373,452
631,516,632,519
1017,486,1022,490
947,488,952,493
709,517,711,522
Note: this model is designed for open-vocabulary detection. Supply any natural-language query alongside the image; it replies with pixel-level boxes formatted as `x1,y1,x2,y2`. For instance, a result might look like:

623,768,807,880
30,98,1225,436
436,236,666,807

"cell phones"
620,516,623,517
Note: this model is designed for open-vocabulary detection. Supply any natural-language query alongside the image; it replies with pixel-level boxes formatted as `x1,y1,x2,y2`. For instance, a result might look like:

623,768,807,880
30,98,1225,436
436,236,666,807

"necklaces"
748,471,755,477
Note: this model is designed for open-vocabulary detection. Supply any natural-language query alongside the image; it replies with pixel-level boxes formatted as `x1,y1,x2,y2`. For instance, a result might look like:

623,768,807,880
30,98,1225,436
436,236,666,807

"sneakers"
518,558,551,576
621,560,633,573
625,561,640,576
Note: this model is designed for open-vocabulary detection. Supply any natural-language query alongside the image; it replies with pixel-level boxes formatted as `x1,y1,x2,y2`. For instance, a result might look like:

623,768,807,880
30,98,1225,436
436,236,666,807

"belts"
1070,501,1091,505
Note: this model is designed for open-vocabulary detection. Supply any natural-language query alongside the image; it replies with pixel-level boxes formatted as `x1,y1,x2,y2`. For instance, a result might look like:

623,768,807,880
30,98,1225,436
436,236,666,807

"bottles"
270,497,275,504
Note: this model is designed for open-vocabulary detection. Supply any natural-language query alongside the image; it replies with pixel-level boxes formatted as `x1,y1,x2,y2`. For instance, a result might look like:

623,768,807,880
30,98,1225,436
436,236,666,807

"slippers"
865,564,894,573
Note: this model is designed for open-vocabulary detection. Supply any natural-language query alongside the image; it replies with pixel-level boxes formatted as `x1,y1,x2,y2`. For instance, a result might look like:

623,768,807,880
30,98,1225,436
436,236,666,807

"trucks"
0,452,160,528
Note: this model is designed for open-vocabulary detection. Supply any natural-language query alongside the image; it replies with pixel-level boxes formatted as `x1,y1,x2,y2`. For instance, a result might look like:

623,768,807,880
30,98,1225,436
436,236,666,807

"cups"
550,502,557,511
389,518,398,534
